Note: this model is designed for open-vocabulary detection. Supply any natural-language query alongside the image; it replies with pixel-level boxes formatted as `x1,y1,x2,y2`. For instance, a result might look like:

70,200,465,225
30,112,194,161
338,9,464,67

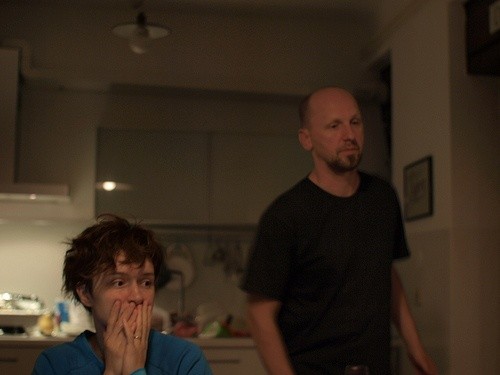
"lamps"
110,10,171,53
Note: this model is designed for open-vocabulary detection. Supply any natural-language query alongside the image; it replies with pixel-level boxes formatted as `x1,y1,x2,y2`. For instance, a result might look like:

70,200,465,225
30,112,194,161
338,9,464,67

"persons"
236,85,437,375
33,212,212,375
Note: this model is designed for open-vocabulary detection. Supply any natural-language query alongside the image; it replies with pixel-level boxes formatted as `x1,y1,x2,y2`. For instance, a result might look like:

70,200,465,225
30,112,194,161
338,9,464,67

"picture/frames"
403,155,435,222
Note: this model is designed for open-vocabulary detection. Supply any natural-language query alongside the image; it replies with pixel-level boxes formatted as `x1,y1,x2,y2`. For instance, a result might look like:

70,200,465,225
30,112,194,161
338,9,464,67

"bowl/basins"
0,311,40,329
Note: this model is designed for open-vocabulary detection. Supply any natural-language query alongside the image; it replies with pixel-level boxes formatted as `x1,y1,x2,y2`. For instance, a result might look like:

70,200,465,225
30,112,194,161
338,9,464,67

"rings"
133,335,142,340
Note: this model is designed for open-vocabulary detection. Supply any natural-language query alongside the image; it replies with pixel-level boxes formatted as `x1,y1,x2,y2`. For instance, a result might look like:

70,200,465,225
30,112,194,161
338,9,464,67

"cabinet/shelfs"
92,124,314,237
